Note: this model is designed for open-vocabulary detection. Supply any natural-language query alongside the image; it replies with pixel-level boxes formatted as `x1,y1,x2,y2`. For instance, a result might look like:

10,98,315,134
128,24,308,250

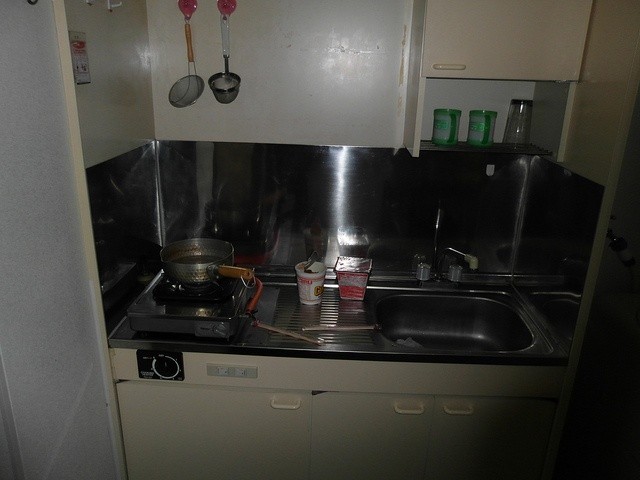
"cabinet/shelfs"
406,0,593,157
100,344,310,480
311,354,566,479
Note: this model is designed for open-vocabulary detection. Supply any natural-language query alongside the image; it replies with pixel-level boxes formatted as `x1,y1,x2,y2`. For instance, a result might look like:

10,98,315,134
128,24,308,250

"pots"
160,237,254,289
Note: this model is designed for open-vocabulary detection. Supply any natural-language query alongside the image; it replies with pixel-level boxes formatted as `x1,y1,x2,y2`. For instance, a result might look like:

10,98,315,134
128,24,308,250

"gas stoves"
126,269,262,338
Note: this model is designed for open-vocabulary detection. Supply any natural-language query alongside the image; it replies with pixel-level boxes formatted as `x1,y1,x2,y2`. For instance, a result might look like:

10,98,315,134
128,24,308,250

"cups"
295,262,326,306
466,109,498,146
432,107,462,146
502,99,532,151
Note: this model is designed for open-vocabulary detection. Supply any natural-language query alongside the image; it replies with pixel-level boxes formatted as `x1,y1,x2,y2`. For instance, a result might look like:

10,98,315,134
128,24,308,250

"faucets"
433,208,441,269
435,247,480,272
